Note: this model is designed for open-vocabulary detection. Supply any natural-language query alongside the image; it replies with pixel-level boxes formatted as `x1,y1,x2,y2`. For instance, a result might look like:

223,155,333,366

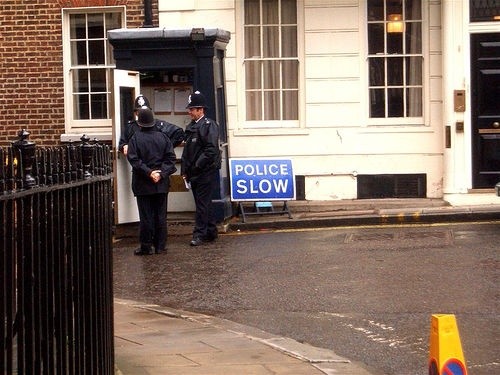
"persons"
180,95,221,246
118,96,185,155
127,106,177,256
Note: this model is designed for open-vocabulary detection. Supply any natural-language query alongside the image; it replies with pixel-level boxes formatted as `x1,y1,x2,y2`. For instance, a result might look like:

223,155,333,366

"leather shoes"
155,247,168,254
134,247,153,255
190,237,202,245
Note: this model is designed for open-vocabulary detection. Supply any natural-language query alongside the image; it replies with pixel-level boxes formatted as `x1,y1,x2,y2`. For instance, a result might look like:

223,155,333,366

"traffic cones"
428,312,467,372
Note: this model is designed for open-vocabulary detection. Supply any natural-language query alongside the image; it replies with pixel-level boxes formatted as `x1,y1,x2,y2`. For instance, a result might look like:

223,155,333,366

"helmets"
185,91,208,108
137,106,155,127
133,95,152,112
